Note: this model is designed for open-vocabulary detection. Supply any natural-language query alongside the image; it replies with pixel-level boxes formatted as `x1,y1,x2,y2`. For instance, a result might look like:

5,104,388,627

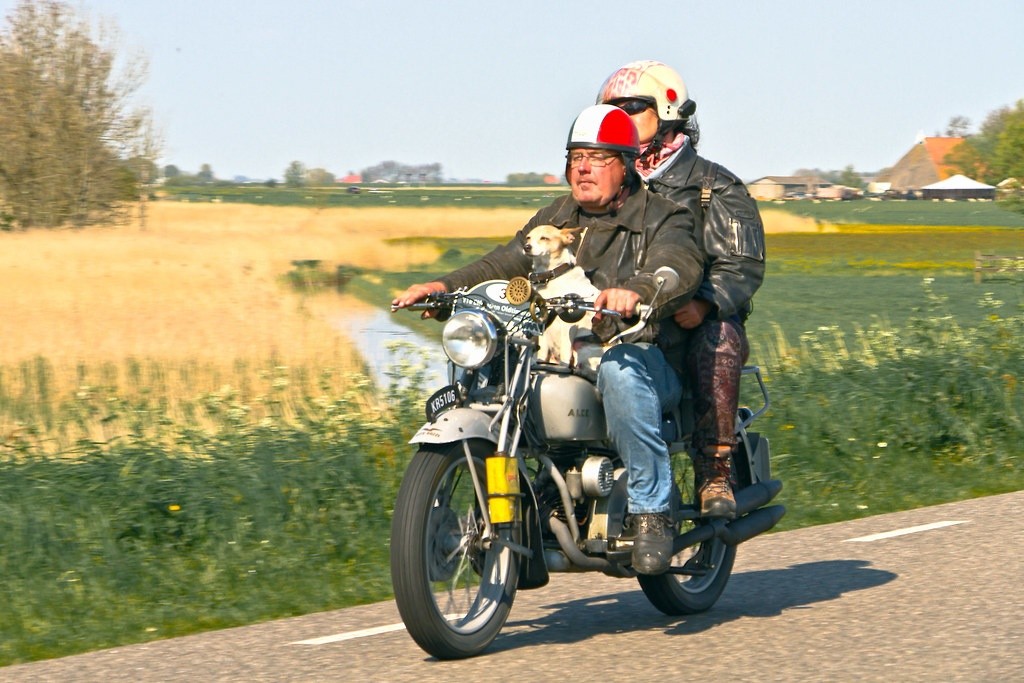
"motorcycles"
391,278,788,659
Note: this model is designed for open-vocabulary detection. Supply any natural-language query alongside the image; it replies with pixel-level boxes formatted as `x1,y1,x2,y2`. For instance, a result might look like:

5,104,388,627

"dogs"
521,223,620,381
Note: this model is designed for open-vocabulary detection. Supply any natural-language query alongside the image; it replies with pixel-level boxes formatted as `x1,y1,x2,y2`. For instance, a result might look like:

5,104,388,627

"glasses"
618,99,649,116
566,155,619,167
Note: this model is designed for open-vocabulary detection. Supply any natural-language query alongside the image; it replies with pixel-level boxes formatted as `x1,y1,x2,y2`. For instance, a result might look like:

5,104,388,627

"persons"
596,60,765,516
389,103,703,573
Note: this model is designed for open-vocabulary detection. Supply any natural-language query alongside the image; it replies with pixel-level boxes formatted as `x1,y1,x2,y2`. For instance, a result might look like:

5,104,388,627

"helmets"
595,59,696,134
564,104,641,157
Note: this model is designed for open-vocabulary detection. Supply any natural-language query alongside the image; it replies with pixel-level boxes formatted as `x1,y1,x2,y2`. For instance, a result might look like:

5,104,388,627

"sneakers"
630,508,675,575
692,446,738,520
